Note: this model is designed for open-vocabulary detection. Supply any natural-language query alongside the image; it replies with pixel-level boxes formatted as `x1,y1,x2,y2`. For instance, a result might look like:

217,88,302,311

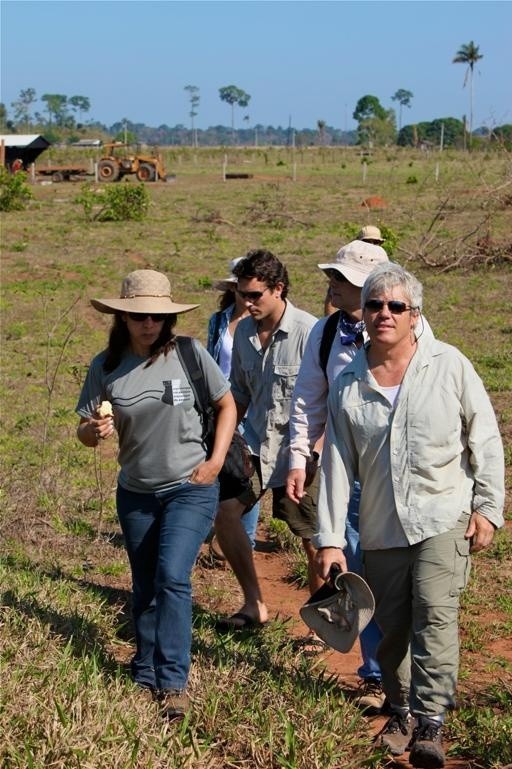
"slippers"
216,613,270,632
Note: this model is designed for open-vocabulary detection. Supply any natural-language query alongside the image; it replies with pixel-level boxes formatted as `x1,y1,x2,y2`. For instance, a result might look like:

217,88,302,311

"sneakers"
407,717,446,768
377,709,419,756
136,687,188,719
354,681,387,714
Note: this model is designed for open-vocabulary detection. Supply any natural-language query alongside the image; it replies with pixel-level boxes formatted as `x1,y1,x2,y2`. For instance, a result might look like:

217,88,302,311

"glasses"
237,288,269,300
127,312,165,323
363,298,415,314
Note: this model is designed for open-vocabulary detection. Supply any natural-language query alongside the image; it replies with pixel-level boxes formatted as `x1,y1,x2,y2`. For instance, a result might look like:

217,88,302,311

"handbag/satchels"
202,405,257,483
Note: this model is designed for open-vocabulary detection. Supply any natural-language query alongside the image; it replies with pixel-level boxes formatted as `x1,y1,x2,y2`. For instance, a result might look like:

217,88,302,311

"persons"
72,270,239,714
213,248,331,657
324,225,386,316
285,239,434,710
205,256,259,556
311,259,507,769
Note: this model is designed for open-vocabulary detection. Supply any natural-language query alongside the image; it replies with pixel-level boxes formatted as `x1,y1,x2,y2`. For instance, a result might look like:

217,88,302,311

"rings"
95,433,99,438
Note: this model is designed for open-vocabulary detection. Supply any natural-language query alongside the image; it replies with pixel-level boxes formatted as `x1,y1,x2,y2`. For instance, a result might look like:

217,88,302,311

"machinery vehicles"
97,141,167,182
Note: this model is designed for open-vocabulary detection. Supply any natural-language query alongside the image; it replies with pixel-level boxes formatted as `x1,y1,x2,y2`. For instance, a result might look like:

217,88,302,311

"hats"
316,240,393,289
299,563,376,654
214,256,248,290
358,224,386,244
91,269,200,316
